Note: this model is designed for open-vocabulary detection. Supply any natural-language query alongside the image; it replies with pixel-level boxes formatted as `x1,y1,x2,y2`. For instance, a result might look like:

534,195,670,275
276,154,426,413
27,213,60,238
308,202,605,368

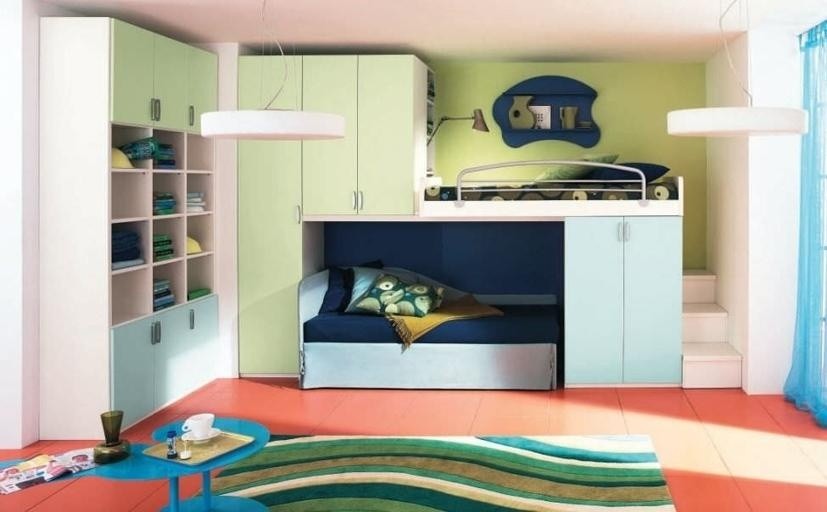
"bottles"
180,435,192,459
167,430,177,458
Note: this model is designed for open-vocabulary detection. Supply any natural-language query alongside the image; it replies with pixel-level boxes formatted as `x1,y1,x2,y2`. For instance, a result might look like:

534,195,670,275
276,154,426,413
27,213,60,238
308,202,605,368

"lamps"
199,1,345,139
429,108,490,150
666,1,809,136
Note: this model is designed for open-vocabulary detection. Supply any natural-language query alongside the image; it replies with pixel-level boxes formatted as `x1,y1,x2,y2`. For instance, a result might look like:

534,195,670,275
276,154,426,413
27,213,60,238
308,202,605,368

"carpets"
191,434,675,512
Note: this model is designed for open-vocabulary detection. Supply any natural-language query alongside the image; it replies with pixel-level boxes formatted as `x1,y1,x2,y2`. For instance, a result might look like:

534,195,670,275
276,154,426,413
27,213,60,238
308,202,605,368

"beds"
425,160,685,217
298,270,560,392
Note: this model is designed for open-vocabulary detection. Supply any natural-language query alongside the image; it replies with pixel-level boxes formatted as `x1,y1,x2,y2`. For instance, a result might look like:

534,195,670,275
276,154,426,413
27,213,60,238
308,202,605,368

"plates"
182,428,220,443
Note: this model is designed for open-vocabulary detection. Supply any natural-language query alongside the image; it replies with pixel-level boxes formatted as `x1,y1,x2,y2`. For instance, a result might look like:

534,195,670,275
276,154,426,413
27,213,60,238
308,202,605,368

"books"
153,278,176,312
0,447,101,496
153,190,175,215
153,142,176,169
153,234,175,263
187,192,206,213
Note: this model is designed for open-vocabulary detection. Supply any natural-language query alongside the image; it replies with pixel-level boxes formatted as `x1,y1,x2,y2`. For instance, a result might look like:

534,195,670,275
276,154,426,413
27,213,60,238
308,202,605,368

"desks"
0,417,272,512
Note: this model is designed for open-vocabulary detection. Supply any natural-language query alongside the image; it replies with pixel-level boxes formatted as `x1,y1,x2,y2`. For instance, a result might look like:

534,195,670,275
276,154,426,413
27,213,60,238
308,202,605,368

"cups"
182,413,215,438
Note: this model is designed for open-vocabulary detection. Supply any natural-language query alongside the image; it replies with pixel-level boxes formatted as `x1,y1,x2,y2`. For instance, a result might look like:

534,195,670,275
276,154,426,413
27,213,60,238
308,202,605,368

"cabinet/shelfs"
301,53,437,216
564,217,683,389
239,53,301,382
493,75,600,148
40,17,220,443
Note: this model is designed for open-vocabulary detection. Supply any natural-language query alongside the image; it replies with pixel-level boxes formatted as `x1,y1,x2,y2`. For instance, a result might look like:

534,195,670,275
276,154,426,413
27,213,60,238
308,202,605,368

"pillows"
357,273,435,317
345,267,385,314
319,265,352,313
